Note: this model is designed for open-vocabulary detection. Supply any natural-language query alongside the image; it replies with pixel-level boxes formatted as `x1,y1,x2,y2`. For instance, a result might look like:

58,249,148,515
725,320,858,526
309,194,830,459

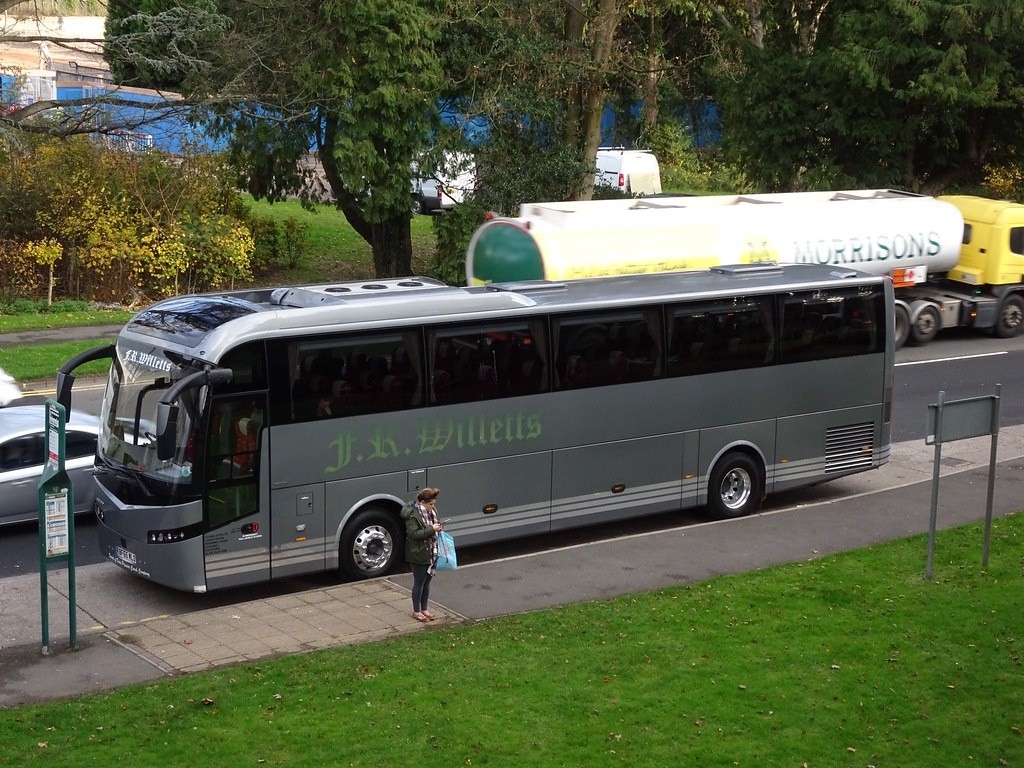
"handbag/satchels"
435,525,457,571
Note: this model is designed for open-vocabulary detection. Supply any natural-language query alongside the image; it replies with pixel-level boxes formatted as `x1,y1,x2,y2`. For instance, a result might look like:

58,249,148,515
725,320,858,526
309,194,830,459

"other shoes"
412,613,430,622
420,611,435,619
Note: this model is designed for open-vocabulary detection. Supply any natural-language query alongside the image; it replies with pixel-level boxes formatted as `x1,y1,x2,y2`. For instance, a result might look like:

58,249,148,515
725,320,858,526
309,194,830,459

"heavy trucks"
469,187,1024,353
408,144,663,218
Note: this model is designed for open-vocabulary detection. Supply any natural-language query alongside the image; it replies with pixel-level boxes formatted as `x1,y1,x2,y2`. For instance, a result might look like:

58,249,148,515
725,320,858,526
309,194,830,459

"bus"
55,263,897,597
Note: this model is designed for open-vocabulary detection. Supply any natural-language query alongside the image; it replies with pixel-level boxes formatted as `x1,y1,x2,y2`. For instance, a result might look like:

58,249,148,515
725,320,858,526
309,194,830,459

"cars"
0,405,156,535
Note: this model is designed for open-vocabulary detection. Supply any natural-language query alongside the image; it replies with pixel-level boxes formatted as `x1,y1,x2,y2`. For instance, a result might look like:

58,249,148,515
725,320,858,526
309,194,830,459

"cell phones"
441,519,451,524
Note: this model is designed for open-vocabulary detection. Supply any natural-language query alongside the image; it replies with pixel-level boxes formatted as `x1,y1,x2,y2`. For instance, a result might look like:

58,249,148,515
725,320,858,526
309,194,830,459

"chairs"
221,418,259,474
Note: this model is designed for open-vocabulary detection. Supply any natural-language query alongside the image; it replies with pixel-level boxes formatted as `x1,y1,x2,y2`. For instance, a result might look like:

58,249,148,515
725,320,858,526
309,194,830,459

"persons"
401,488,443,622
